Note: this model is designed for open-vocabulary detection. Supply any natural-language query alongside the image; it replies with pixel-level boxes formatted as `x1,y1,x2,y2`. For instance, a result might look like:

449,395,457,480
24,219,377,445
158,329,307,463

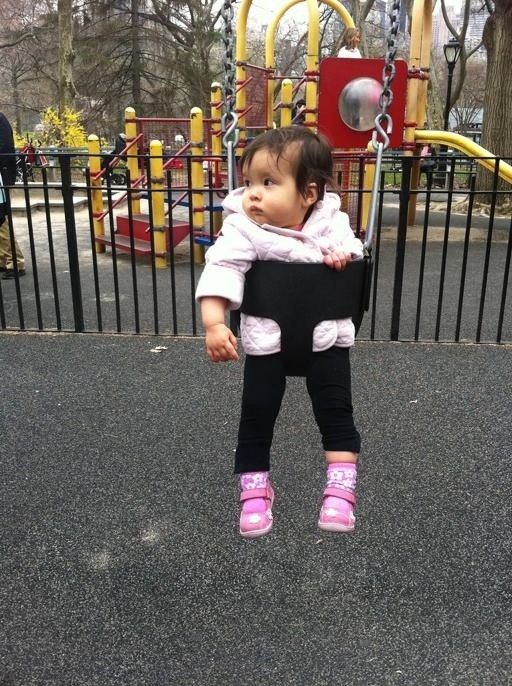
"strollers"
100,148,126,185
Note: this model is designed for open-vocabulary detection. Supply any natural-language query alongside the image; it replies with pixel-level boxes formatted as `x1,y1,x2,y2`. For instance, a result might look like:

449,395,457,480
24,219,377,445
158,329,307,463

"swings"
231,0,401,377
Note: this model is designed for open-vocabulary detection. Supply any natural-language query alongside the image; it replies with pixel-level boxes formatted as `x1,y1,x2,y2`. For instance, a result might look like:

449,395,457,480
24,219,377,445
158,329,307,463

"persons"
191,122,369,538
0,111,25,279
419,143,436,190
99,132,128,170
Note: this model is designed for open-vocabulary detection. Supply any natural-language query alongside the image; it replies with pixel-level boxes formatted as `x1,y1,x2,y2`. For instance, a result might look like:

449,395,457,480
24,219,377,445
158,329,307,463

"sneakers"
0,267,25,279
318,482,356,532
239,480,274,538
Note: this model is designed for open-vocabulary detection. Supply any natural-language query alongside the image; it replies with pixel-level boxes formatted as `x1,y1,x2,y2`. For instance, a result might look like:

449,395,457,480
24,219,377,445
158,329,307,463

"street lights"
435,37,461,185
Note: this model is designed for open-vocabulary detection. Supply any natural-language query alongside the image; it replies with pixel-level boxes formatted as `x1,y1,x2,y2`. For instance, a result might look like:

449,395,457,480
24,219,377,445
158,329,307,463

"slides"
403,129,512,184
